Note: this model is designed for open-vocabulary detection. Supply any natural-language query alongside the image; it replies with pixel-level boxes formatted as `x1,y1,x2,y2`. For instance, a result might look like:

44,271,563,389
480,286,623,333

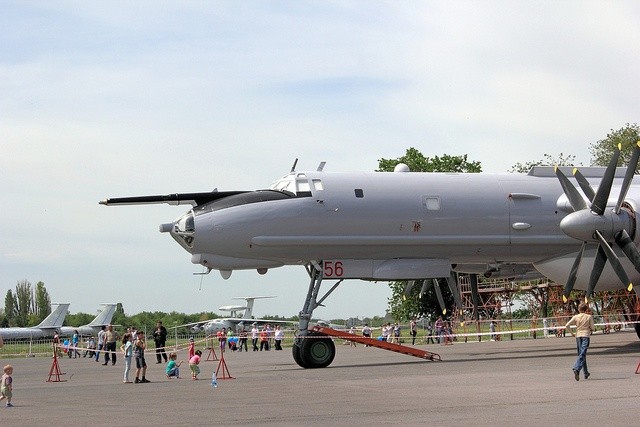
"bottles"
212,371,217,388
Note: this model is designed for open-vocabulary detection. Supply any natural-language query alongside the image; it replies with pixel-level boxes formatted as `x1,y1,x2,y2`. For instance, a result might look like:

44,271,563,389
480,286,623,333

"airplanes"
61,303,122,340
99,140,640,369
167,295,299,338
0,303,70,344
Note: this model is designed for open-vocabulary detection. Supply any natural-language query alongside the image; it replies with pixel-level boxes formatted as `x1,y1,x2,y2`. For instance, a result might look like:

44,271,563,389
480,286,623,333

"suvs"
317,318,332,324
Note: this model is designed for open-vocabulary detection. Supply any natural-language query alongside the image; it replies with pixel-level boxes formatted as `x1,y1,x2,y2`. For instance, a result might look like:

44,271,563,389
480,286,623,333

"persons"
489,320,496,341
386,322,392,344
67,340,72,358
51,329,62,357
103,324,117,366
188,337,194,361
362,324,372,347
90,337,98,359
189,349,202,381
427,321,434,345
82,337,90,357
565,303,596,381
443,320,454,345
72,330,81,361
121,332,132,384
382,325,387,341
410,317,416,345
435,315,443,343
153,321,166,362
393,322,402,344
95,325,109,363
348,326,357,346
0,365,13,408
131,326,138,340
216,324,285,351
132,330,147,384
122,326,131,343
165,352,183,379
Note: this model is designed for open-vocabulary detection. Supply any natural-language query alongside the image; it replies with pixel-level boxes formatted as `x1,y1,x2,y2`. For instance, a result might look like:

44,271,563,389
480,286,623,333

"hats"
189,337,194,341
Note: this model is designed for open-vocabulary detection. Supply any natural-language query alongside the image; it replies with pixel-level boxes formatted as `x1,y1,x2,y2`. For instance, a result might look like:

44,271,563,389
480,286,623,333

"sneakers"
573,370,579,381
112,362,115,365
140,378,150,382
584,372,590,379
135,379,142,383
6,402,12,407
102,363,107,365
